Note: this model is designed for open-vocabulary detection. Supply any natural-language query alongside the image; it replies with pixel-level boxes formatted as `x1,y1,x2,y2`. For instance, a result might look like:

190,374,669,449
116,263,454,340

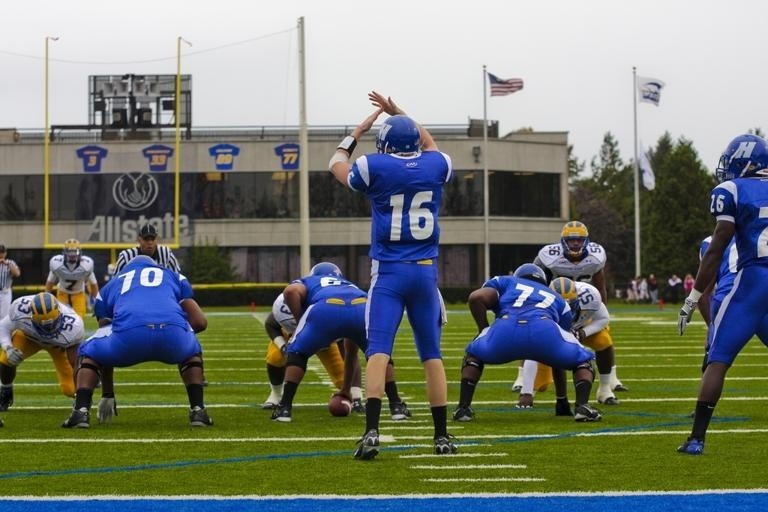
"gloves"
556,397,572,416
97,392,118,425
678,297,698,336
4,345,24,366
515,393,534,409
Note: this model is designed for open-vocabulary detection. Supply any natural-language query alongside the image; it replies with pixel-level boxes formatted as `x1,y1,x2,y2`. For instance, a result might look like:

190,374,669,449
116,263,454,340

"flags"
486,74,523,98
637,73,665,109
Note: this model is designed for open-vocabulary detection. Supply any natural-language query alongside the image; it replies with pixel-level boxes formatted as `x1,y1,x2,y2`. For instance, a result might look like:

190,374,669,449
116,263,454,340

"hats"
138,222,158,240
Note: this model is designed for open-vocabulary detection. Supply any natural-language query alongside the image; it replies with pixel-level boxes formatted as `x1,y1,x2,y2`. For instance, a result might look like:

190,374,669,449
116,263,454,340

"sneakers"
433,432,459,454
188,404,214,426
389,399,412,419
574,384,628,420
262,402,292,422
0,386,14,413
351,398,366,414
61,403,90,428
353,428,379,460
675,438,704,454
452,402,474,422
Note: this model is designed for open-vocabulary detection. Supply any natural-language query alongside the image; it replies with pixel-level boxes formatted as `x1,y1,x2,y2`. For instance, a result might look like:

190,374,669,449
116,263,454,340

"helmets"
63,239,82,265
376,114,421,154
548,277,578,304
126,255,157,266
560,221,589,263
512,263,547,286
31,292,65,340
309,262,342,277
716,134,768,184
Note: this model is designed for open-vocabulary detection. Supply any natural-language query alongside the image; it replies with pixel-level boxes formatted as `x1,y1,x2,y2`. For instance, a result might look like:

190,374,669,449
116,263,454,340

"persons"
0,244,20,323
452,262,603,423
257,280,366,414
696,220,739,385
92,263,117,317
510,220,630,396
514,275,618,412
44,238,100,324
675,135,766,457
61,254,215,429
0,291,86,431
269,260,413,424
109,225,210,389
624,273,696,306
326,89,462,460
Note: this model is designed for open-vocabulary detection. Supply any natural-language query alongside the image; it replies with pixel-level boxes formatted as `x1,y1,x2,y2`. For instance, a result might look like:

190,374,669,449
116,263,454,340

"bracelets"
687,288,704,307
337,134,358,157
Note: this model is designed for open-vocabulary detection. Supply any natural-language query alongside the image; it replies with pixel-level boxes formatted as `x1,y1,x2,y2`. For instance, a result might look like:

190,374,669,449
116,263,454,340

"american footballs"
329,395,352,416
